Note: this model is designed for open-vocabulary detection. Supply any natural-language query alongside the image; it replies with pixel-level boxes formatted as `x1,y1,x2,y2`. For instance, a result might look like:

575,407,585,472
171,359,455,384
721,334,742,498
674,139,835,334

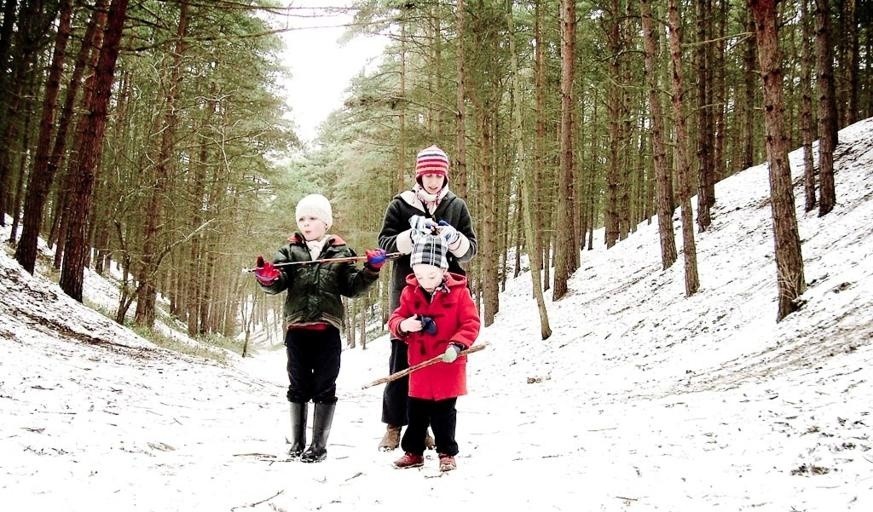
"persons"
257,194,386,464
378,145,480,471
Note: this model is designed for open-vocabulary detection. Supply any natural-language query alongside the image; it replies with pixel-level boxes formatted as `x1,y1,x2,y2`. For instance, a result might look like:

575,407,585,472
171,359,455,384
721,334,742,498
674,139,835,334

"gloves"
444,345,460,363
255,256,281,286
400,313,423,332
396,215,470,258
365,248,386,272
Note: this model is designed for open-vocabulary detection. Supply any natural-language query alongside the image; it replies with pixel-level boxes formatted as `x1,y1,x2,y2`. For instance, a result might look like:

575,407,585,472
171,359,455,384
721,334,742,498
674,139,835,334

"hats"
410,236,449,268
416,144,448,184
296,194,333,231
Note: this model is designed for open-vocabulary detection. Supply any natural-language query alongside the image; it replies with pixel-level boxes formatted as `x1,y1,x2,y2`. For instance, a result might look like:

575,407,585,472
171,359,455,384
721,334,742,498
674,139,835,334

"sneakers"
378,424,456,471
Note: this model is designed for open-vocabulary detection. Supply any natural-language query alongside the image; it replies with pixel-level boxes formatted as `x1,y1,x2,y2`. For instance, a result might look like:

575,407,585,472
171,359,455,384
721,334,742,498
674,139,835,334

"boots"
288,401,336,462
